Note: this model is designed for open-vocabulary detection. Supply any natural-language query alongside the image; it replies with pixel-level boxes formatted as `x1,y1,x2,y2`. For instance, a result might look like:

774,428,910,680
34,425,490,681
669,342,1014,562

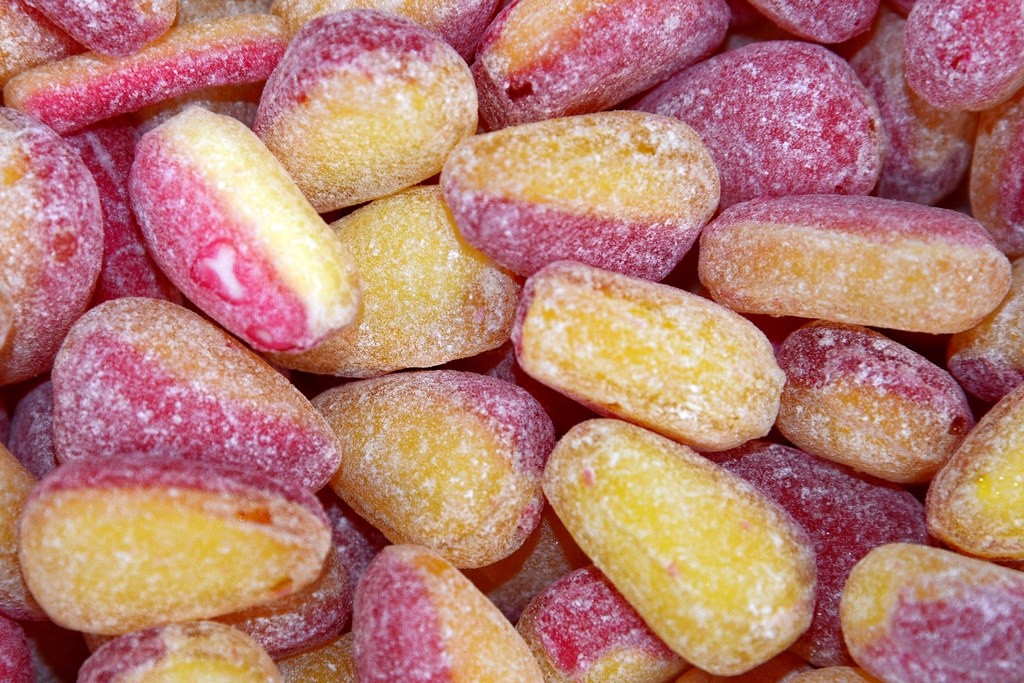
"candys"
0,1,1024,683
